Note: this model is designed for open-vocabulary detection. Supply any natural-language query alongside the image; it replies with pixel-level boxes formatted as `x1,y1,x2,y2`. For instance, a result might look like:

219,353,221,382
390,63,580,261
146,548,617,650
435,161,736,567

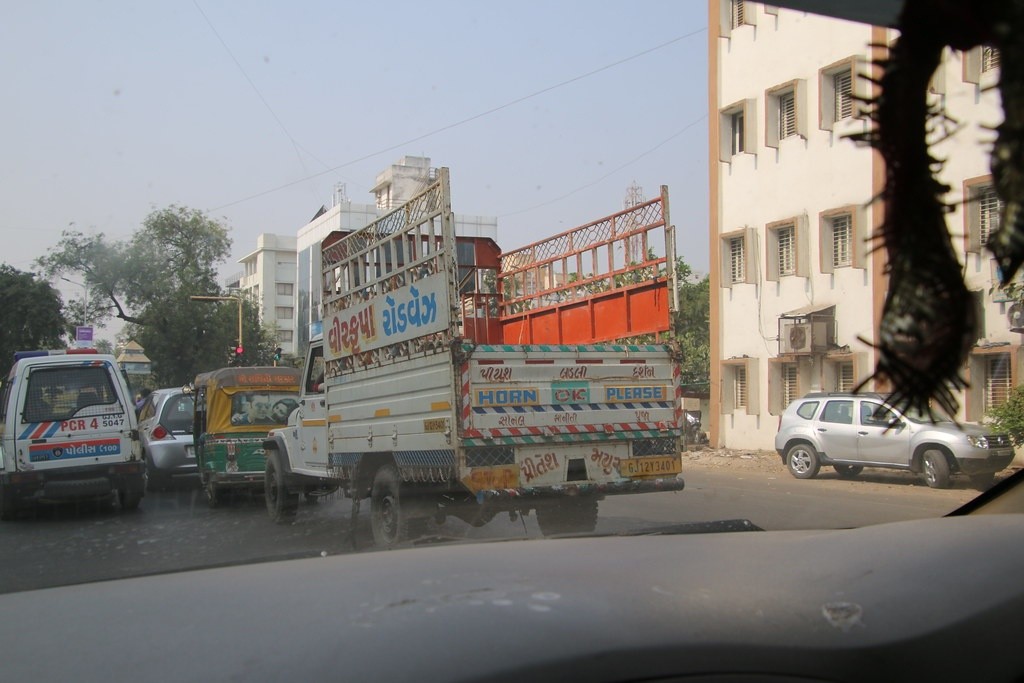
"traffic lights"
273,348,282,361
235,347,243,357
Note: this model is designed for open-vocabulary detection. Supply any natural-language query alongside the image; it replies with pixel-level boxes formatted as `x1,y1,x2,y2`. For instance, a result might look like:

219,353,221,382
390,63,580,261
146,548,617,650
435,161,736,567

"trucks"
262,167,687,550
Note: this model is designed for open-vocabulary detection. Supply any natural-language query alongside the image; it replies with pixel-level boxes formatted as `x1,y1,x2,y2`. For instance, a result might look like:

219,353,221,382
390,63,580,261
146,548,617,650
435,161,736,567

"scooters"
182,366,303,508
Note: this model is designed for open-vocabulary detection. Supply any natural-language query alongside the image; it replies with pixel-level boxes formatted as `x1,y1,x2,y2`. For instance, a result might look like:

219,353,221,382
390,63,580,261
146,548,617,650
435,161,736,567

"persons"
233,392,278,425
135,388,150,408
231,398,299,425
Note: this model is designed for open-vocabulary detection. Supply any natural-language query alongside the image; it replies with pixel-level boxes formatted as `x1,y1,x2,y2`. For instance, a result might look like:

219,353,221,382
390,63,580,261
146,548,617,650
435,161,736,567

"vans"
0,349,142,522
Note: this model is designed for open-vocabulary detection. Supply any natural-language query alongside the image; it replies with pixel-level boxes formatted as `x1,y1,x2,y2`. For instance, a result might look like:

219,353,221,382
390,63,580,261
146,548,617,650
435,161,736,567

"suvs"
773,392,1015,488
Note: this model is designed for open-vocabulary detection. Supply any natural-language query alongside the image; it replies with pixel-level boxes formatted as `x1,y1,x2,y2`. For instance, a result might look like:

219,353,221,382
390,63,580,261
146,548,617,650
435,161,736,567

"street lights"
190,294,244,367
61,278,88,326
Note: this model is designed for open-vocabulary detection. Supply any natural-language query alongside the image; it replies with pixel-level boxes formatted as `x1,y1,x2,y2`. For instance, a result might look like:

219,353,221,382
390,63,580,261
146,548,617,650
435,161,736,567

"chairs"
861,406,875,425
25,386,51,423
69,392,105,418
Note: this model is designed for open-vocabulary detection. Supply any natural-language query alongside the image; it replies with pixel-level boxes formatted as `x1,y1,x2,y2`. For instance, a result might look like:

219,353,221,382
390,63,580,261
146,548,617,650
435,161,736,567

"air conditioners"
784,322,834,353
1004,301,1024,342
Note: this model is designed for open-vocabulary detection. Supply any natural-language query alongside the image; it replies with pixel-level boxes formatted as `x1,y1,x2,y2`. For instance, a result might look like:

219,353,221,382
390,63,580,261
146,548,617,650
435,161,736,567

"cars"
138,387,200,494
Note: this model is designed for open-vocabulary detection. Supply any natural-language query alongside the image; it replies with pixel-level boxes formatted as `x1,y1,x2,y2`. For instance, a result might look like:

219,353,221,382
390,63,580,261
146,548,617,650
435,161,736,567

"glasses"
256,402,271,409
